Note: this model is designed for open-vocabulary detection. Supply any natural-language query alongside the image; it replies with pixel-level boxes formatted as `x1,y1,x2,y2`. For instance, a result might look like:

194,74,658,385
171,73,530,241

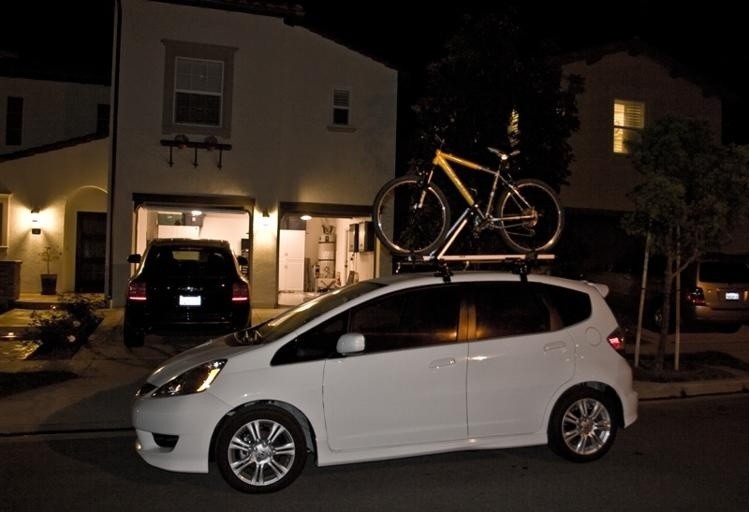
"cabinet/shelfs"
277,229,305,292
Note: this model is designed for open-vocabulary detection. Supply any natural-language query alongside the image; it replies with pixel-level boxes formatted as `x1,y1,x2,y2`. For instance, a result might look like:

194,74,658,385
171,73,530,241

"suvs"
129,252,645,499
122,234,256,354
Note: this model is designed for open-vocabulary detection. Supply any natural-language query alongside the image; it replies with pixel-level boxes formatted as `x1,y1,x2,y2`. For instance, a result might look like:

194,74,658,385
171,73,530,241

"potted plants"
38,245,62,295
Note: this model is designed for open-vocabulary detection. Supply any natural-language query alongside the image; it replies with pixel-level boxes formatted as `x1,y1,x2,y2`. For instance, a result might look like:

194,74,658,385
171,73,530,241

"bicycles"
370,105,569,261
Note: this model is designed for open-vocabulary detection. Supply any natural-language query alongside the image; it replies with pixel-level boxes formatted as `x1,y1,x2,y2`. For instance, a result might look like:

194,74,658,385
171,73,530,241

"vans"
584,249,749,336
